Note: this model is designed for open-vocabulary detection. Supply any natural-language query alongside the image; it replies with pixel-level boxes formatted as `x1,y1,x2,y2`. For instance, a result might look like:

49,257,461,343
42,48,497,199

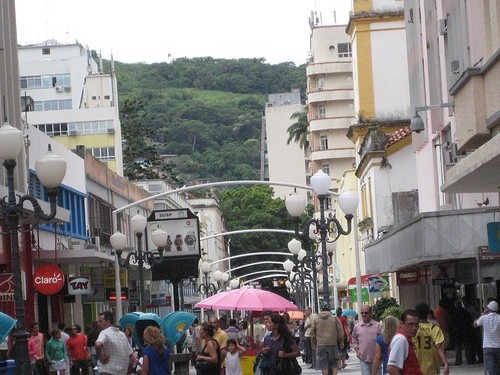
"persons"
6,301,500,375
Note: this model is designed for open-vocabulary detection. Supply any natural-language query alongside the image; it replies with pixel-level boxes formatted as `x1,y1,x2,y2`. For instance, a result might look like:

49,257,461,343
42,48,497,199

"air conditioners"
47,129,115,138
55,86,62,91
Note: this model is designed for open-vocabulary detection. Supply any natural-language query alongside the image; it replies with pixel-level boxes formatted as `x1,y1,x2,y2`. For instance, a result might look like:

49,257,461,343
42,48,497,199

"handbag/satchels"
196,341,216,370
46,363,49,372
254,354,264,375
270,333,302,375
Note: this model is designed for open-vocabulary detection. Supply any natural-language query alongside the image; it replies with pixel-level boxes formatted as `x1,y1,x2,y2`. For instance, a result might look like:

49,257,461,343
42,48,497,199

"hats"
320,301,330,310
487,301,498,311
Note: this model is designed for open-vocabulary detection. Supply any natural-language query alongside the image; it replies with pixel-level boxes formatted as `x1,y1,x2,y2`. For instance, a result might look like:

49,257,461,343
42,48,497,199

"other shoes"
341,364,347,368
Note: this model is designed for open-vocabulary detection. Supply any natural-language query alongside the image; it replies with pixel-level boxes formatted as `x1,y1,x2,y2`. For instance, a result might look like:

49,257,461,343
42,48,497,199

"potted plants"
356,216,372,233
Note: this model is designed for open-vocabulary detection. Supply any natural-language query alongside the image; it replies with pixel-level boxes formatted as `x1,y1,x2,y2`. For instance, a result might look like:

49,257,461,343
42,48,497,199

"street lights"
0,120,69,375
197,258,228,323
282,168,359,313
110,213,167,313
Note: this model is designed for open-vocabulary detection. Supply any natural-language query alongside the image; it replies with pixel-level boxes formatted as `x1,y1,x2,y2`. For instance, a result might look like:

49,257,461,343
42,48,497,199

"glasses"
360,312,368,314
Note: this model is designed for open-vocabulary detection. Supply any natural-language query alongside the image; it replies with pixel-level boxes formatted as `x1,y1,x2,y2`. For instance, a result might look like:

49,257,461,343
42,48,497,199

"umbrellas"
195,286,299,334
284,311,305,320
